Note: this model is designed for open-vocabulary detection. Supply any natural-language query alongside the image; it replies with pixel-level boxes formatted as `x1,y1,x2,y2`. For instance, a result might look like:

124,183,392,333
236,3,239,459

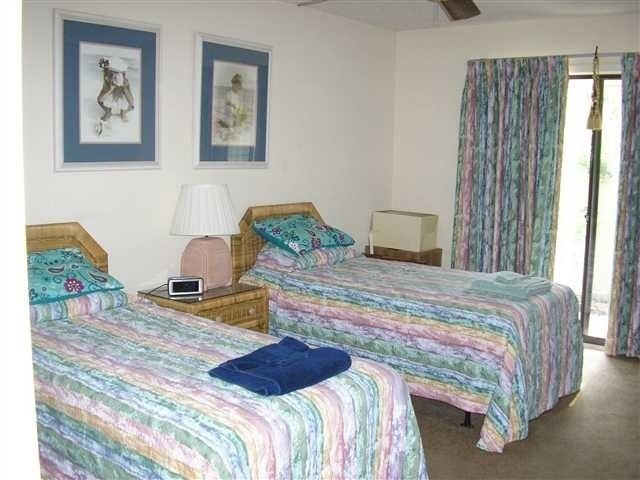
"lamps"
171,183,241,292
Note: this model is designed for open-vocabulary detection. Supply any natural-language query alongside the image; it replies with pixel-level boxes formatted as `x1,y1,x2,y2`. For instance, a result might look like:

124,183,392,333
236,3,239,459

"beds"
230,201,584,453
25,222,430,475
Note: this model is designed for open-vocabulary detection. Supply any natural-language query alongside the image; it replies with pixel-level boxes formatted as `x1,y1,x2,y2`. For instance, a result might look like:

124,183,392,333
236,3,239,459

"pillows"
259,236,360,273
249,211,355,255
29,289,128,324
27,246,124,307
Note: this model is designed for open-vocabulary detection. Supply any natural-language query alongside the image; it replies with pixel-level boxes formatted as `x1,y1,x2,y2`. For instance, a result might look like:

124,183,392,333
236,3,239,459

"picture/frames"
192,32,275,169
51,8,161,171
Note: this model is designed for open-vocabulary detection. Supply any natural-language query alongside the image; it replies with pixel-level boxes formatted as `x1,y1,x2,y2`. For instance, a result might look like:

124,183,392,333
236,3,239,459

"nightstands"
138,281,268,334
364,244,442,268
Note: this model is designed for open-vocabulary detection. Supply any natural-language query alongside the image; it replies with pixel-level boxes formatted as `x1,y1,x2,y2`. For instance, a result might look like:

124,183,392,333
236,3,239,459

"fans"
440,0,481,21
